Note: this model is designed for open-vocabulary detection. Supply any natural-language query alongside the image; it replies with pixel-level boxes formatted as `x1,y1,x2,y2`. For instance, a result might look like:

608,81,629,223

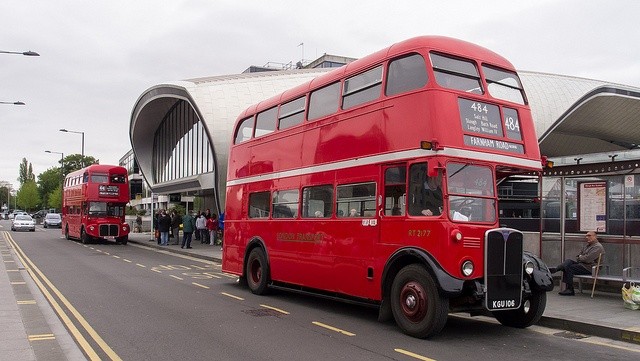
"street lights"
1,102,27,105
60,129,85,169
45,150,64,177
0,50,39,57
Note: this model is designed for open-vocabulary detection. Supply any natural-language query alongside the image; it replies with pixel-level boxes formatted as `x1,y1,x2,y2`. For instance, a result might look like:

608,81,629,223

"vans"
31,210,52,219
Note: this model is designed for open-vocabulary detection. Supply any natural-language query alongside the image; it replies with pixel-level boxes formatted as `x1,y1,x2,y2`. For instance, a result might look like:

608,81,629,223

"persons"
392,208,402,216
205,214,218,245
180,211,195,248
219,212,225,251
136,215,142,232
154,214,159,244
338,210,344,218
548,231,606,295
315,211,322,218
414,171,443,216
157,212,169,246
194,211,201,240
205,209,211,220
195,212,208,244
170,211,180,245
348,208,359,217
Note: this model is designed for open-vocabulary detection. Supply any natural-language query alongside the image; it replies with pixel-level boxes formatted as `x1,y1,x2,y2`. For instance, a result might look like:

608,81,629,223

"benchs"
558,264,639,295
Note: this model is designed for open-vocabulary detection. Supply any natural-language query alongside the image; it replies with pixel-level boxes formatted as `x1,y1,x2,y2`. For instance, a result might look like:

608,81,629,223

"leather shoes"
559,289,575,295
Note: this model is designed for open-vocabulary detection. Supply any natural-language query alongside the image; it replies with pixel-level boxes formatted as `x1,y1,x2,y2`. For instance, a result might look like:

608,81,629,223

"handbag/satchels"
155,229,159,238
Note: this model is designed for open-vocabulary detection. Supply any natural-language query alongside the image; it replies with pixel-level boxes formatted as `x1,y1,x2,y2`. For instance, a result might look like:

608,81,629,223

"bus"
62,165,130,245
222,36,553,337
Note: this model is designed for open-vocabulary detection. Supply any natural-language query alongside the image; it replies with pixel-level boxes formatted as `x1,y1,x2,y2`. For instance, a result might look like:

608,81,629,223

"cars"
43,213,61,228
8,210,29,219
11,215,35,232
